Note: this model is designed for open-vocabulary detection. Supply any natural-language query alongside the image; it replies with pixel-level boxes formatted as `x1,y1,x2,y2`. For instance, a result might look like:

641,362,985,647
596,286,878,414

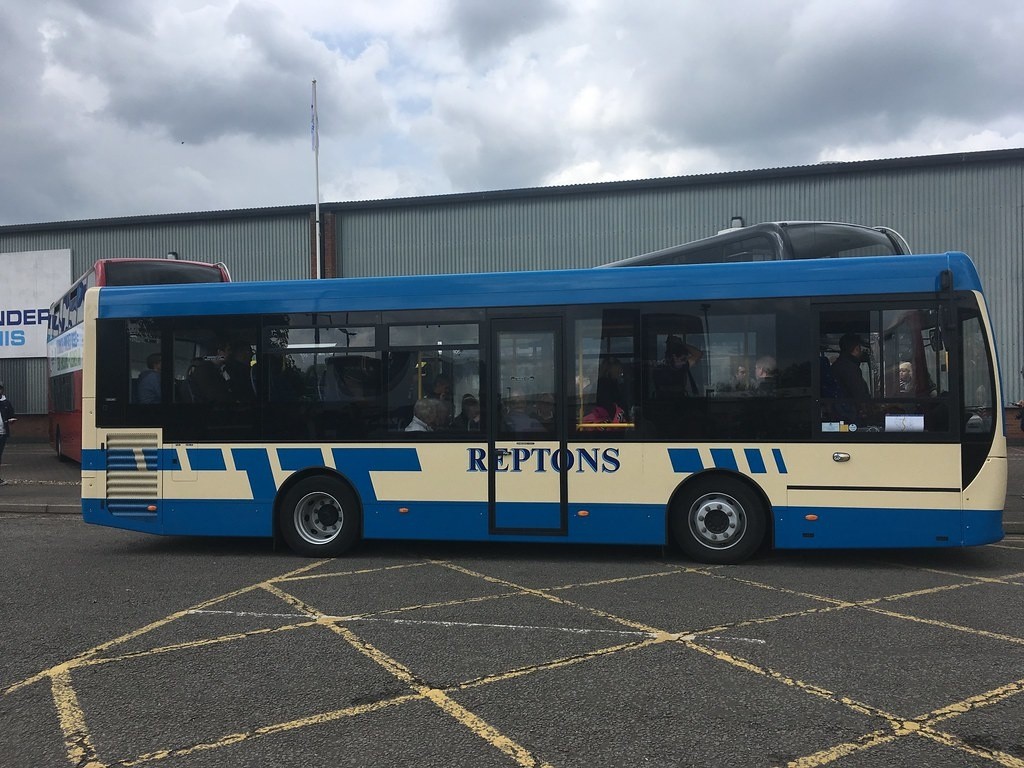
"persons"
191,341,255,404
404,366,557,432
976,374,990,417
0,382,16,486
831,335,870,418
653,336,704,396
724,365,755,392
581,356,634,431
899,362,912,390
754,357,776,391
137,354,172,405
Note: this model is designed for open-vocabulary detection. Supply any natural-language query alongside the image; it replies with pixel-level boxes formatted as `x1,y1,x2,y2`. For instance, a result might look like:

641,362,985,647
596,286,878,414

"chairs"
186,364,196,404
250,362,258,397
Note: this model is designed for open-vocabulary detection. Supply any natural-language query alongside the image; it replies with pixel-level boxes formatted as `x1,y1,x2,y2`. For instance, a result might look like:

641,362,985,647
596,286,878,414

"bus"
557,219,913,398
46,257,234,463
81,250,1009,565
